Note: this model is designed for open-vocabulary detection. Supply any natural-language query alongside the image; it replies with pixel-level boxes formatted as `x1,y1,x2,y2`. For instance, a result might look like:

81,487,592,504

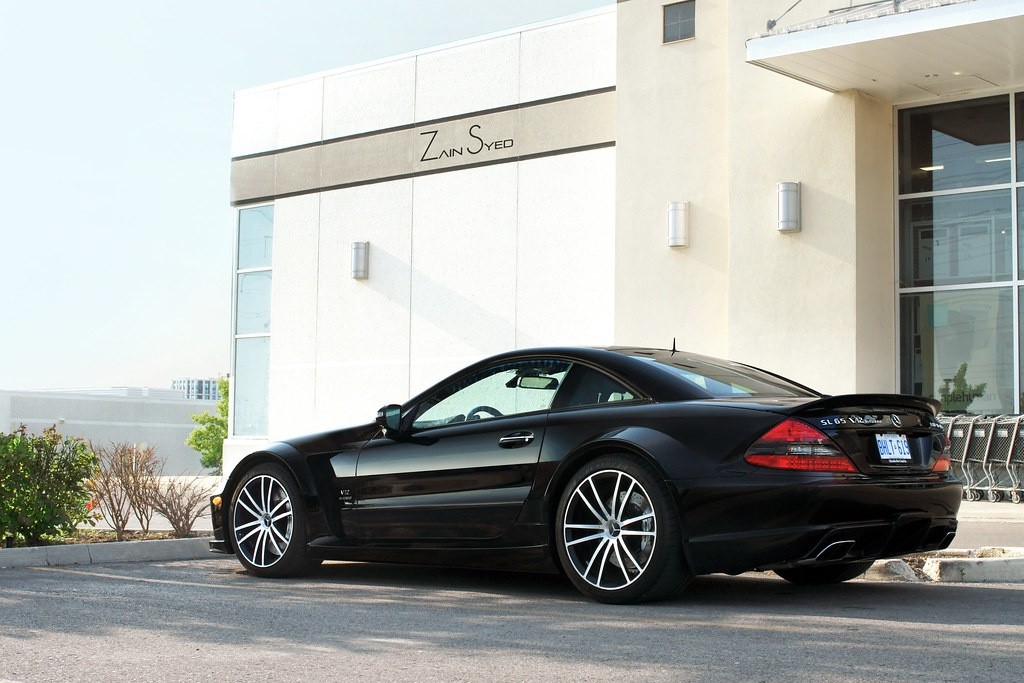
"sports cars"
205,344,964,607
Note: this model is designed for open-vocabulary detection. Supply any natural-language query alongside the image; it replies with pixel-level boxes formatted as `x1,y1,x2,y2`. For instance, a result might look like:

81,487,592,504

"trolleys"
926,414,1024,503
